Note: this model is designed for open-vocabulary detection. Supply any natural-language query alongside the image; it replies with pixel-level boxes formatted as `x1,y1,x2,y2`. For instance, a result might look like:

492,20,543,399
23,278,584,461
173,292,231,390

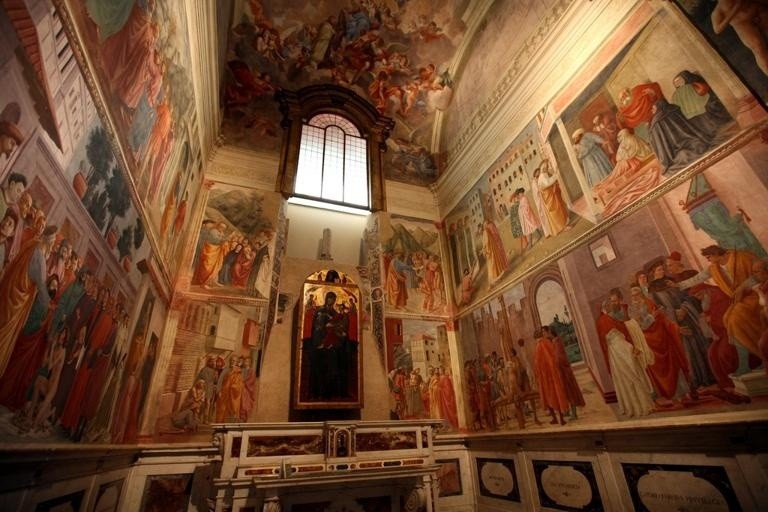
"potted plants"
71,125,116,200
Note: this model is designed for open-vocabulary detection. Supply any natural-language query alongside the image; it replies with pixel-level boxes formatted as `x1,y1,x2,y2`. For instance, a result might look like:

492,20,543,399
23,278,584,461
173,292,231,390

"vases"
124,256,133,273
107,228,119,249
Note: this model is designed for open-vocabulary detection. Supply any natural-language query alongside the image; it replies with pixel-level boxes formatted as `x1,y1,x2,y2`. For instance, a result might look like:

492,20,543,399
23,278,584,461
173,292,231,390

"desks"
492,392,542,428
210,418,443,512
